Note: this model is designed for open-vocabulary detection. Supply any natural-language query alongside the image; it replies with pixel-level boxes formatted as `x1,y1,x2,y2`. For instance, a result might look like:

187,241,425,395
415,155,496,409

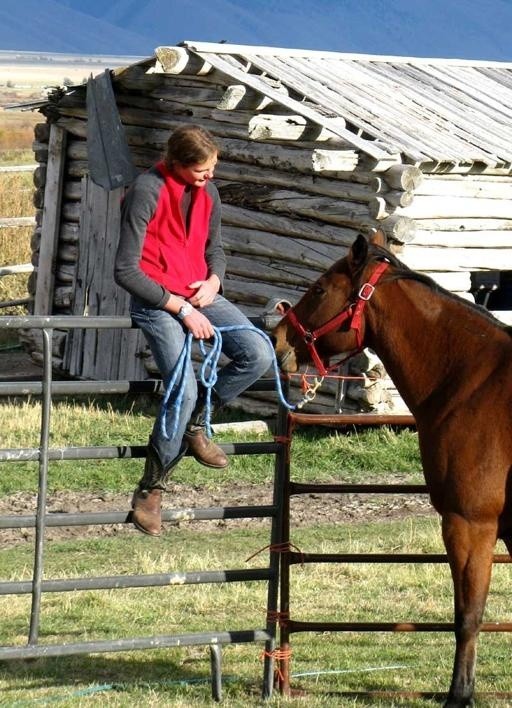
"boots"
183,381,230,468
131,434,189,537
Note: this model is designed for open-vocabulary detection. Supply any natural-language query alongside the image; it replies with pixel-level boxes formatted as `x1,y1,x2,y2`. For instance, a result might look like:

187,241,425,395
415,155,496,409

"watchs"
177,300,194,320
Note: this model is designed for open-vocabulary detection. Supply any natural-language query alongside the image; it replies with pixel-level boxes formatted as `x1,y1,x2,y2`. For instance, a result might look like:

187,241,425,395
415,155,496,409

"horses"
269,225,512,708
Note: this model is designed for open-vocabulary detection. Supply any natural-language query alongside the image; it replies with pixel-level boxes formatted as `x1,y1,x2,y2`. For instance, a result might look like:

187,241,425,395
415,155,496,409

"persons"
113,122,273,538
